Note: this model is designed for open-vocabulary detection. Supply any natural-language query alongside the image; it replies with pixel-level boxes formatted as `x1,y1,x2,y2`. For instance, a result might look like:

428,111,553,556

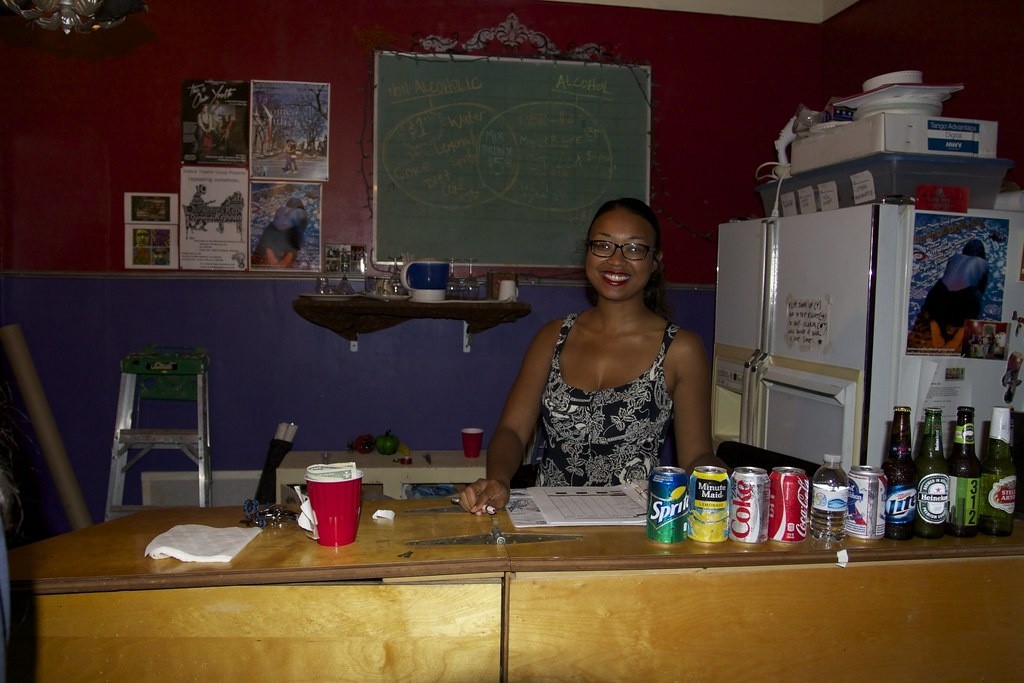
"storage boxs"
756,155,1015,209
487,271,519,300
789,112,997,175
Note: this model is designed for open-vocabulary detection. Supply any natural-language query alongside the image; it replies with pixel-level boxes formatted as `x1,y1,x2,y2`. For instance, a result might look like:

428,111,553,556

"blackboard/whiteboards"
372,49,651,268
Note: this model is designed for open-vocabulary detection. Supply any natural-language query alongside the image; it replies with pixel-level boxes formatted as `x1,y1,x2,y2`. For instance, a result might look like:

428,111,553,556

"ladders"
102,342,208,523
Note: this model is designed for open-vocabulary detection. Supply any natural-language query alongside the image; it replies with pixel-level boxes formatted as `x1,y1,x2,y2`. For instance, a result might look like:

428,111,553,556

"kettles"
400,258,450,302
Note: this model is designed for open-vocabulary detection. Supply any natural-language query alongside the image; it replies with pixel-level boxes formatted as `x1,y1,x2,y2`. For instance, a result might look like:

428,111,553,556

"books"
506,477,652,527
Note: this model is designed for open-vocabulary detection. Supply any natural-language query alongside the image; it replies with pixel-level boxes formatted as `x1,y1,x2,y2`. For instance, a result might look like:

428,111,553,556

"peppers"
375,430,399,454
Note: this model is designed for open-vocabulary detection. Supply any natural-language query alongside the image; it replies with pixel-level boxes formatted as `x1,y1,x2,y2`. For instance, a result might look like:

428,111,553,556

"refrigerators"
708,208,1024,471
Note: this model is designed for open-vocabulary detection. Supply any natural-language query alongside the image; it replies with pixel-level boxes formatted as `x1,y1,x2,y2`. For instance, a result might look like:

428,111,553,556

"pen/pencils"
450,496,497,514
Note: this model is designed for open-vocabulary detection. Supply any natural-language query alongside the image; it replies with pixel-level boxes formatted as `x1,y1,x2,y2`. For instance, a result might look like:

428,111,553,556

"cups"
461,428,484,458
303,470,364,547
498,280,519,302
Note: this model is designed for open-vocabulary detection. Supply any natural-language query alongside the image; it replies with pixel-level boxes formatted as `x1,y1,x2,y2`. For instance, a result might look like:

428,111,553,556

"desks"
5,496,1024,683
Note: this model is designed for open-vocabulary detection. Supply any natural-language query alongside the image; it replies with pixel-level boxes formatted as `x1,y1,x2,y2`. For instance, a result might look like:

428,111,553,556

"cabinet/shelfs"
275,451,487,505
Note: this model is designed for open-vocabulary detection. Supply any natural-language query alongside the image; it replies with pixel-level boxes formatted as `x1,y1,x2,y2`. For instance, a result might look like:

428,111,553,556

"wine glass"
460,258,480,300
447,256,461,300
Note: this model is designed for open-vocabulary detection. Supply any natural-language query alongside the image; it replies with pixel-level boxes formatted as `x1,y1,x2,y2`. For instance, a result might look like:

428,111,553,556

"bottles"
946,406,983,538
913,407,949,538
981,406,1017,538
880,405,918,540
809,455,849,543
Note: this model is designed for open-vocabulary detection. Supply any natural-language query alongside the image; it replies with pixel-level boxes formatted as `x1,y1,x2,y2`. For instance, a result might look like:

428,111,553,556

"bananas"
397,443,410,457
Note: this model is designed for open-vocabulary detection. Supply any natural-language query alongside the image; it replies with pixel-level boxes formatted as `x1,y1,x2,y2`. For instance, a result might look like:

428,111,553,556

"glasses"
587,239,656,262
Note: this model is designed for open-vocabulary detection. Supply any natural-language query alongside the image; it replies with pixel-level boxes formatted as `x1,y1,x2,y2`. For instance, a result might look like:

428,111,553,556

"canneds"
768,466,809,543
730,466,771,544
687,466,731,542
846,465,888,540
646,466,690,544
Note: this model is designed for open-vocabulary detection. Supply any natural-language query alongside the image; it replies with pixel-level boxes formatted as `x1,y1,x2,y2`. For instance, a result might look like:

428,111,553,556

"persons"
457,198,732,517
911,238,989,348
282,139,298,173
194,104,236,160
250,197,309,269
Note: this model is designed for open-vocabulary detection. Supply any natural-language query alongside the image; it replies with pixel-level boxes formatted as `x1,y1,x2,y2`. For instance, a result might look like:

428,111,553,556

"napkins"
143,525,263,564
